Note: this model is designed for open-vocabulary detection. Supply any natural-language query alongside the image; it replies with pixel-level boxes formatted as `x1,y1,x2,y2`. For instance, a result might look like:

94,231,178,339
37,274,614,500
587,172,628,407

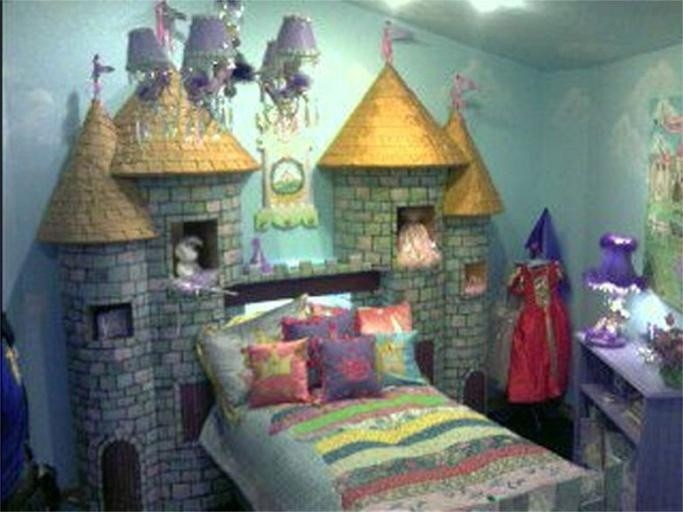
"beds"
198,379,604,512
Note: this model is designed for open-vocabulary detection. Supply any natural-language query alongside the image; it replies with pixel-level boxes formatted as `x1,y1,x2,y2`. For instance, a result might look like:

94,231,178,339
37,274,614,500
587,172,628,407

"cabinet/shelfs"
571,325,682,512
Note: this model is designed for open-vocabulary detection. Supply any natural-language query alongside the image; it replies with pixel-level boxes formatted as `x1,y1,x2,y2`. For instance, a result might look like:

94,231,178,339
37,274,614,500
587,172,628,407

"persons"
0,302,68,512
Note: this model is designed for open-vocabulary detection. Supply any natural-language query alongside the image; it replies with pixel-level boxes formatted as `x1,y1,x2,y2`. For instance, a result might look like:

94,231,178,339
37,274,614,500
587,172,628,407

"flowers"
636,313,682,369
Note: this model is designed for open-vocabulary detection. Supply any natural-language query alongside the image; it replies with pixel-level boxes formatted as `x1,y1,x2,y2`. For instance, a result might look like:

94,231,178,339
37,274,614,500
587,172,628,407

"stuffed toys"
172,236,203,281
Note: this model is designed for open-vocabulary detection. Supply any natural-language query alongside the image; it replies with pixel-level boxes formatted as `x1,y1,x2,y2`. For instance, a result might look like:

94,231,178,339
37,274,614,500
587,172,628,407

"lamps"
126,13,319,118
580,232,646,350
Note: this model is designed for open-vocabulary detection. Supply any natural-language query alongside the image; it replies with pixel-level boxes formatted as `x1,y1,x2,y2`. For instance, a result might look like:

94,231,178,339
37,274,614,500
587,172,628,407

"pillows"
194,293,430,414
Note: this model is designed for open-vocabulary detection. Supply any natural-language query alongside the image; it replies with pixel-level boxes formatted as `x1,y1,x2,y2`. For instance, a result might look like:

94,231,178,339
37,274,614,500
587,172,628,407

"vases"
657,365,682,389
